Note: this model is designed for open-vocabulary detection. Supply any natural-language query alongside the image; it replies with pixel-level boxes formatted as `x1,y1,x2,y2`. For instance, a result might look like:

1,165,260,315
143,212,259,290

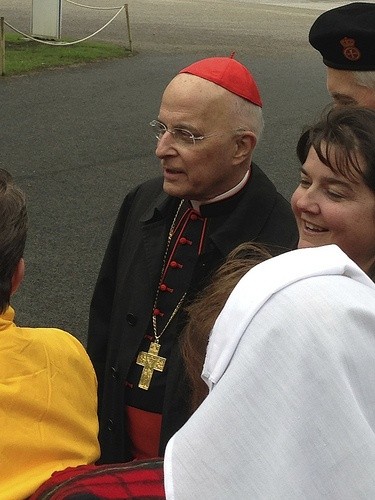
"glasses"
149,120,242,148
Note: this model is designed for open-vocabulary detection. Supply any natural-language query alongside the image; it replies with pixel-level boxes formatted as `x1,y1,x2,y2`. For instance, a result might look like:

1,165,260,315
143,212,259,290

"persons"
289,106,375,284
308,2,375,112
163,243,375,500
86,52,300,466
0,167,101,500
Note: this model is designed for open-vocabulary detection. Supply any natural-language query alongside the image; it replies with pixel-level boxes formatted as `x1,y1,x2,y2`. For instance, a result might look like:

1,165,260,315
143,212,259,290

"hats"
180,51,262,108
308,2,375,71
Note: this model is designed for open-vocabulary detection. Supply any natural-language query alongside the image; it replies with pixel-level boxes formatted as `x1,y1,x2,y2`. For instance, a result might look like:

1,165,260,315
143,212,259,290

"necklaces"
135,199,186,390
